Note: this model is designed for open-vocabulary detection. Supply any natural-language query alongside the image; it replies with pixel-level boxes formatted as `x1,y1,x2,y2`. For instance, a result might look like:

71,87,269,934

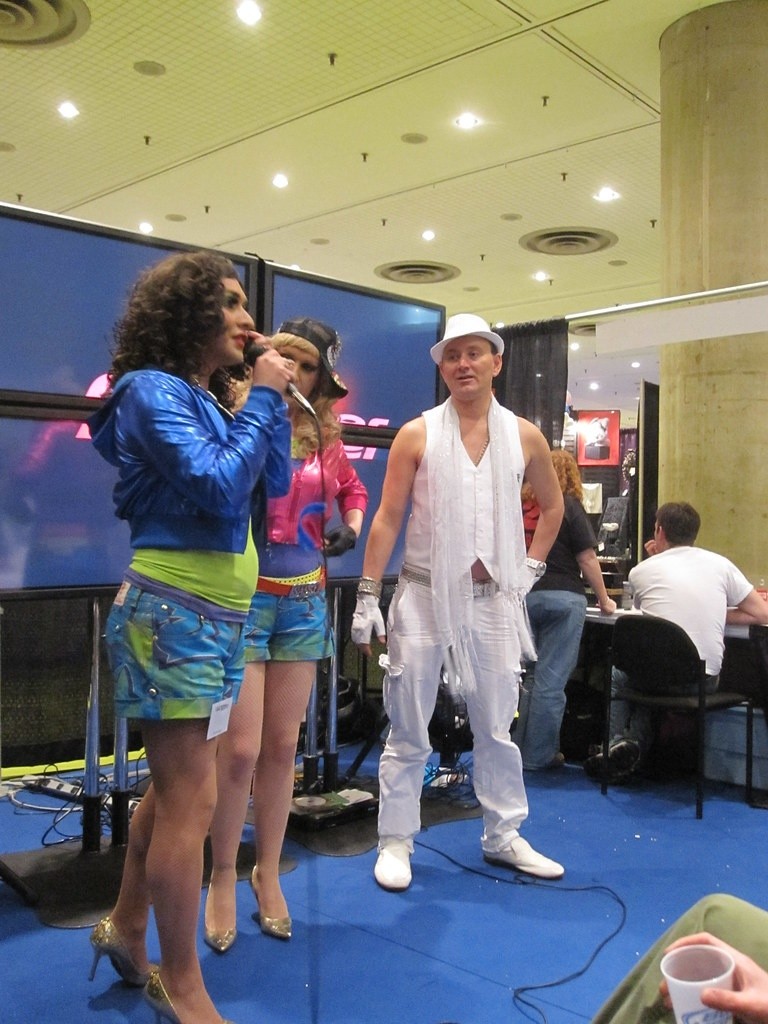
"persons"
94,254,291,1024
203,318,368,952
584,501,768,783
512,450,617,772
351,314,564,888
590,893,768,1024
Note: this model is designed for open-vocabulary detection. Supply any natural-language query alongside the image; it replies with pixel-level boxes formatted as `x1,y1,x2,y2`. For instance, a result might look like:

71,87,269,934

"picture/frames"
574,410,621,465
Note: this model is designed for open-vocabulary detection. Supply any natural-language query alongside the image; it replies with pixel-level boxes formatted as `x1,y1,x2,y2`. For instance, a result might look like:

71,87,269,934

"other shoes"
375,845,412,891
584,742,639,777
484,837,565,878
554,753,565,766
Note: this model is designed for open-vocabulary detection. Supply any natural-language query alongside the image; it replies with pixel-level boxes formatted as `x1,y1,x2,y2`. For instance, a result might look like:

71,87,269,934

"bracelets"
357,577,382,597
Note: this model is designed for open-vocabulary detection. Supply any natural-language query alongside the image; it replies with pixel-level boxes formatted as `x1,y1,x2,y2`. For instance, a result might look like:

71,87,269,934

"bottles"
756,578,768,603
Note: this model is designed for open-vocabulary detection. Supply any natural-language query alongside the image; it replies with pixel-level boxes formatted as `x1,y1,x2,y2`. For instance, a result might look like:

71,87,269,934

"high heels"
251,865,292,939
143,971,235,1024
88,917,159,986
205,871,237,951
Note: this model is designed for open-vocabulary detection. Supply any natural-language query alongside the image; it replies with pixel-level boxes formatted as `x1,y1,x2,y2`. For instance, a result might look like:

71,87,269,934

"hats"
280,316,348,399
431,314,505,365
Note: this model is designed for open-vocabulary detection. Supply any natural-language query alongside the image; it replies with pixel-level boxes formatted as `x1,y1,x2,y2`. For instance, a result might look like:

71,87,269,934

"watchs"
523,558,547,575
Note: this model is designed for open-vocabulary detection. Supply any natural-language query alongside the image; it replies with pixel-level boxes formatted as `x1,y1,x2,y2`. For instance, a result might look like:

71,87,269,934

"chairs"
597,615,755,822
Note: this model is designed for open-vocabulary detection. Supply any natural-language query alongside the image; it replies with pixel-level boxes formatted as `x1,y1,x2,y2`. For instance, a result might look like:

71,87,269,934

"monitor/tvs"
1,203,447,598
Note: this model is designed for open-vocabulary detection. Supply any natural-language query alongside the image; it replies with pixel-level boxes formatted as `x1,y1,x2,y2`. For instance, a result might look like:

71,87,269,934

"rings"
286,359,296,367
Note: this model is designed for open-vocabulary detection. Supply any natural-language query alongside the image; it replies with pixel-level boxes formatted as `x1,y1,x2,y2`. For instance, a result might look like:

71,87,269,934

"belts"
256,569,326,598
401,563,498,597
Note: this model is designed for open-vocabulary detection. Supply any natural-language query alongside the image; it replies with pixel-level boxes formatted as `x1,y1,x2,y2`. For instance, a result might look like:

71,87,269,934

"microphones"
244,345,315,418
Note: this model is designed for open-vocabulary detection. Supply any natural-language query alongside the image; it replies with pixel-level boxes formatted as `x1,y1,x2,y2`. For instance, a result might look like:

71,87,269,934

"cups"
622,581,632,610
660,945,735,1024
